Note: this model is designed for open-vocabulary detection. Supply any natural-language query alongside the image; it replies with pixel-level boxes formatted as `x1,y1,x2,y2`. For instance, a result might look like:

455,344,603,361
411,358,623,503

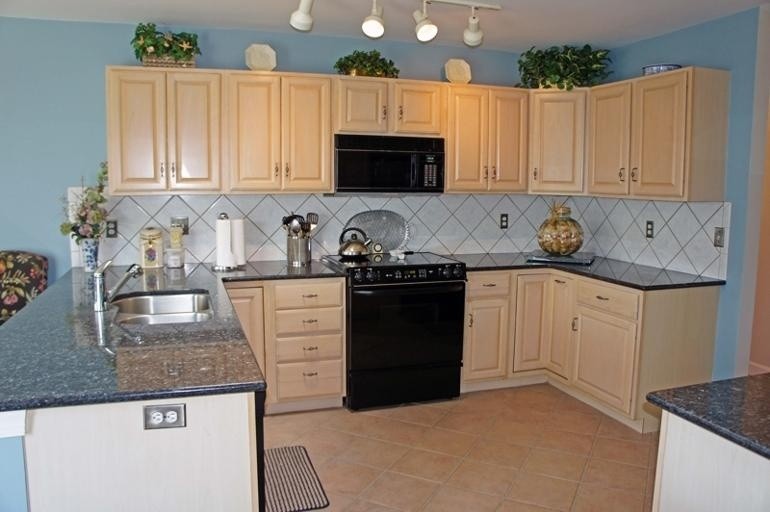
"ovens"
348,282,466,379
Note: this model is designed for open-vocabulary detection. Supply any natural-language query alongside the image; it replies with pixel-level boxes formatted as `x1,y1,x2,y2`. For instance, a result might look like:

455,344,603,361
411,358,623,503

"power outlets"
143,403,185,429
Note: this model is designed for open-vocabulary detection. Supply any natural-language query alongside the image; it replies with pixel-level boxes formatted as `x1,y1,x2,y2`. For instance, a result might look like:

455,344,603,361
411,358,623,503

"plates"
244,43,277,71
443,58,472,84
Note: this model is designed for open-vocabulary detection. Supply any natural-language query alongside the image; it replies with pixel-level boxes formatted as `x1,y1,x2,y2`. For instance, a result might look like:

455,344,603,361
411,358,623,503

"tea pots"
338,257,370,268
338,227,372,257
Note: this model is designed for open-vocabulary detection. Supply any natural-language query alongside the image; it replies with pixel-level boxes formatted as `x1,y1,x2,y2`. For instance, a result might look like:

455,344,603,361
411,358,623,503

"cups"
286,236,313,267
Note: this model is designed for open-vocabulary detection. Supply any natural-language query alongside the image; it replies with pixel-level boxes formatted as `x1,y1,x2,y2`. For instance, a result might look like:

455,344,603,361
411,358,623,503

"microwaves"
334,134,445,194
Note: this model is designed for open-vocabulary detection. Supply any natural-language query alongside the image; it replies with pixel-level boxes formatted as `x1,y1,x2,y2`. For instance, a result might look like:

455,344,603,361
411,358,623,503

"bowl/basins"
389,249,404,256
642,63,683,76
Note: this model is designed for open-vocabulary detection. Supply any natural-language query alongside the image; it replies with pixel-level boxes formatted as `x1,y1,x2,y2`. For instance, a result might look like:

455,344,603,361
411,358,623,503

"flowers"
62,163,110,243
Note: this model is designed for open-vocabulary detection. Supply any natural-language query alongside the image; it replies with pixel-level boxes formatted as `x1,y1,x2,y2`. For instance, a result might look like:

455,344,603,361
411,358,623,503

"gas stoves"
322,251,467,286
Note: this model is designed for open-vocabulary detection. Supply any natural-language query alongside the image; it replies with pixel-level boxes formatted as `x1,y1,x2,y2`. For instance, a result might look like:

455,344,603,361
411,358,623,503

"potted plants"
131,20,202,67
334,47,401,77
513,42,617,92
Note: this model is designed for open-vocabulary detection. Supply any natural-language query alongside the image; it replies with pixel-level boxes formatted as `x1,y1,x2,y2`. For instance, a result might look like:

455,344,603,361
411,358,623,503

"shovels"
301,212,318,238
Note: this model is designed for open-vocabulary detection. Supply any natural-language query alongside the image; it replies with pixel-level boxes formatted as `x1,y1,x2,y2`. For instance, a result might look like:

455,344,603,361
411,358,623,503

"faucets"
94,312,144,362
93,259,144,312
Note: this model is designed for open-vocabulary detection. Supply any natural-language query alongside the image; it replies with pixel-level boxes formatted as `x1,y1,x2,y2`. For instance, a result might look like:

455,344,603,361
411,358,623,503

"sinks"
115,311,214,325
109,288,214,313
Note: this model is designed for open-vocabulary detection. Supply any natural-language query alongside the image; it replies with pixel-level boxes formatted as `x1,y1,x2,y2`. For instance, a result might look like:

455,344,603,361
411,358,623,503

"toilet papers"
230,218,247,266
215,219,238,268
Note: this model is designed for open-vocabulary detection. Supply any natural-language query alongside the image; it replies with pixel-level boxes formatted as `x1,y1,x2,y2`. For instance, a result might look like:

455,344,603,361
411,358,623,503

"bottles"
142,270,167,292
139,226,164,270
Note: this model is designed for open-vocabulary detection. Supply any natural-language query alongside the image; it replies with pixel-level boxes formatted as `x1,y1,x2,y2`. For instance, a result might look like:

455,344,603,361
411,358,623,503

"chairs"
0,250,50,326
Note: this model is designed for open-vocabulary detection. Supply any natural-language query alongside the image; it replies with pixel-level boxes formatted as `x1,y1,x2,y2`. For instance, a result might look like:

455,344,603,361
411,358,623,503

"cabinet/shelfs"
106,66,228,197
541,268,575,403
574,273,719,434
225,281,266,385
461,270,511,393
587,67,730,202
508,268,550,389
261,277,346,414
527,88,589,196
228,69,338,194
442,81,531,194
332,74,447,138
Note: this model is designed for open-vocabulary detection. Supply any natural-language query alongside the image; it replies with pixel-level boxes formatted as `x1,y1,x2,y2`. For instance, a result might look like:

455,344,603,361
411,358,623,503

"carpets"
261,443,329,511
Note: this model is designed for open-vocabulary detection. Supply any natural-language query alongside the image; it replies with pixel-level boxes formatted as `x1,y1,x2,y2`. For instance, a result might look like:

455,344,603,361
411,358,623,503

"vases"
82,239,98,272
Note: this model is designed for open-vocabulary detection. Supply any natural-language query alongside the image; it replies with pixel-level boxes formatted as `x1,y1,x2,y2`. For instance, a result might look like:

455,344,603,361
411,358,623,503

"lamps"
289,0,501,46
